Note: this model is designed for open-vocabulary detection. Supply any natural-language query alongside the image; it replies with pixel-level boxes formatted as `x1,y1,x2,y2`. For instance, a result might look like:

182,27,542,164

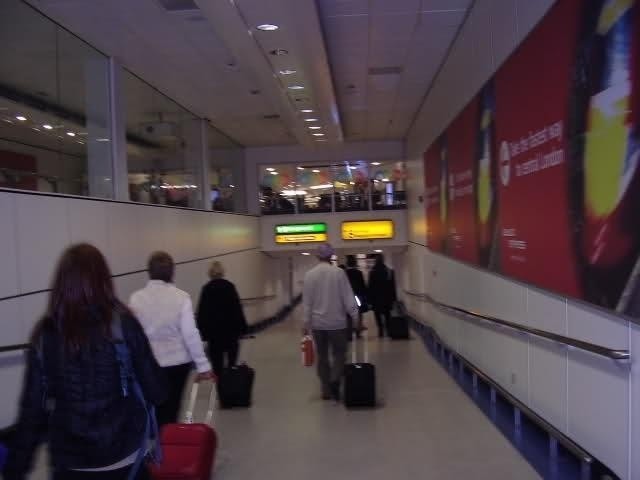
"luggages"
390,317,412,340
343,329,378,409
218,333,256,409
148,379,217,480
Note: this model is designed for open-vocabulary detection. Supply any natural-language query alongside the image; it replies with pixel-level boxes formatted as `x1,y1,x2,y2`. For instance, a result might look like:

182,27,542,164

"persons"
128,250,218,428
299,244,363,403
0,242,160,479
208,183,393,213
366,255,396,338
193,259,256,408
344,256,366,340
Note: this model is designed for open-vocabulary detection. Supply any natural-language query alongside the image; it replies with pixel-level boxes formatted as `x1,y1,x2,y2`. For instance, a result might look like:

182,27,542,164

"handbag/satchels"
300,332,313,367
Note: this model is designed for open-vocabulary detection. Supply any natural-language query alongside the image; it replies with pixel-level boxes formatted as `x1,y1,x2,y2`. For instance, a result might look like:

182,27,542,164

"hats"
311,242,336,258
